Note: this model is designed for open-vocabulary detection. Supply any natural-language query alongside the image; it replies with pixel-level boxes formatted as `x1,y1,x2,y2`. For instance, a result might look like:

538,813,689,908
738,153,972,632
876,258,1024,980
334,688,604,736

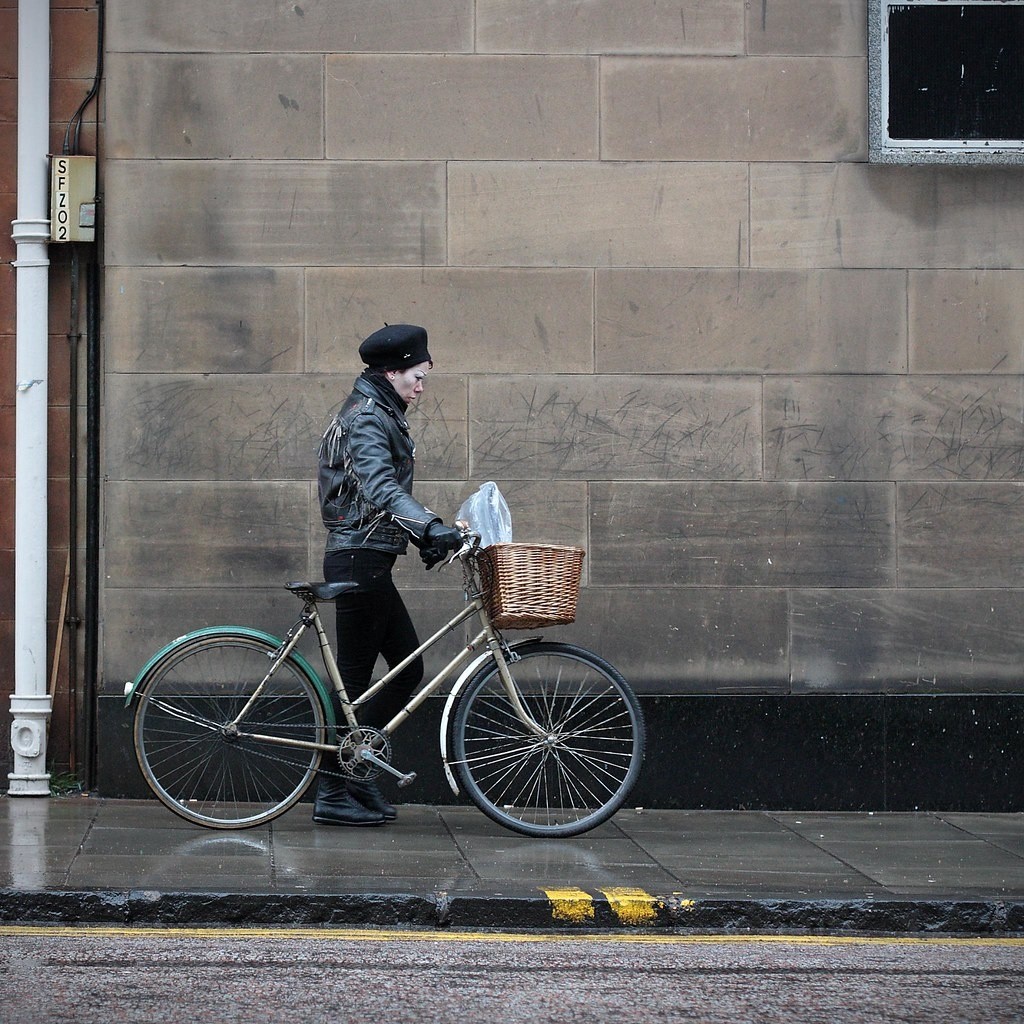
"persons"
312,322,463,826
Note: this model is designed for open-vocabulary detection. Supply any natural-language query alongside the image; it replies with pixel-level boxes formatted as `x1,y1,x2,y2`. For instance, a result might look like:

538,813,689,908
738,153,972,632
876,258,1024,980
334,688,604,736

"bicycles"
120,538,651,839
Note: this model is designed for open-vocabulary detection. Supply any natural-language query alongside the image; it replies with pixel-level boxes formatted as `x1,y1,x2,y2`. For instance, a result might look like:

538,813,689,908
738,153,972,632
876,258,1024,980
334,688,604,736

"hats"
358,321,432,374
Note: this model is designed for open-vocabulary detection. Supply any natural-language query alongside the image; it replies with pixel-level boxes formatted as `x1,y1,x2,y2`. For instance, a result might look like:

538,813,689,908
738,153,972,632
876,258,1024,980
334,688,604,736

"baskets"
475,542,587,630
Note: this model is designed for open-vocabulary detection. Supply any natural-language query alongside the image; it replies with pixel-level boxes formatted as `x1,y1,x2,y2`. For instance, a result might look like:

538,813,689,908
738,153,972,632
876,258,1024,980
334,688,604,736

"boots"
312,768,398,826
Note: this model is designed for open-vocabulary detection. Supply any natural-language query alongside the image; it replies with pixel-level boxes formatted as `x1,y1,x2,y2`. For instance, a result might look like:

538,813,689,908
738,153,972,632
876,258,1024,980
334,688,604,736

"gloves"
418,522,464,570
